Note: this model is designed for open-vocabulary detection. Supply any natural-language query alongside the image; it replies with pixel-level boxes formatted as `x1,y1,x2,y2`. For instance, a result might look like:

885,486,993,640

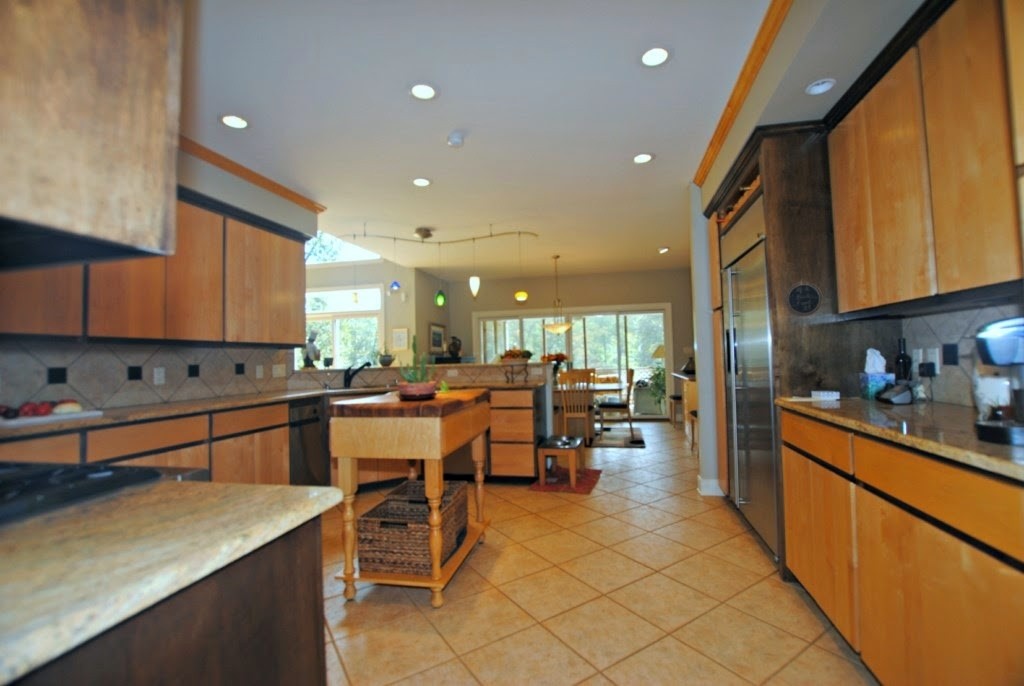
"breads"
53,402,82,413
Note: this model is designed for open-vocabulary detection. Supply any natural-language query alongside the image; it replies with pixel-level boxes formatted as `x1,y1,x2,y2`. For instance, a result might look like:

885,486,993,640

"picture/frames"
428,323,446,355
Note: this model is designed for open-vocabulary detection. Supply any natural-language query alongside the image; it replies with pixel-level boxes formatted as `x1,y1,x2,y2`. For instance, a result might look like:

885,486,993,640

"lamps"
542,255,575,336
650,344,665,360
328,224,539,307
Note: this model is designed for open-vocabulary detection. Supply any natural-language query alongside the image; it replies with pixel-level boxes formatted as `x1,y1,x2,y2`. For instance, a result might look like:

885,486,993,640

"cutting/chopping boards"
0,411,103,427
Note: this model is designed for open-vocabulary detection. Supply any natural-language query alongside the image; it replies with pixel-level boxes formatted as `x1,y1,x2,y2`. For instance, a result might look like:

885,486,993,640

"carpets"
529,466,603,495
586,427,646,448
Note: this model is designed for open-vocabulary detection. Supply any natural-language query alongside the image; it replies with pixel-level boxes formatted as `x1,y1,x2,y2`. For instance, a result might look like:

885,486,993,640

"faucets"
343,361,372,388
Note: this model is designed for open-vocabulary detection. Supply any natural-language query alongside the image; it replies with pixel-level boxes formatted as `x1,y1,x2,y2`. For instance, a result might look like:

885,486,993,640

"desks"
552,384,623,440
672,372,697,445
329,389,492,609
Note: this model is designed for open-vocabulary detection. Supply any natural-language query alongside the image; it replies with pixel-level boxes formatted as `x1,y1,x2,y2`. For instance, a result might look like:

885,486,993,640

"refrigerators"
719,194,783,562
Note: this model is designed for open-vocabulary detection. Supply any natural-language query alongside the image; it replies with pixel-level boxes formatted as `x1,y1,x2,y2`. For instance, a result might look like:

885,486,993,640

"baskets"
357,497,458,576
385,480,469,539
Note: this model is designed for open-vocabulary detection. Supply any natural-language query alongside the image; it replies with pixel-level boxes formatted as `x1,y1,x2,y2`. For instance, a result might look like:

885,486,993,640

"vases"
553,362,559,383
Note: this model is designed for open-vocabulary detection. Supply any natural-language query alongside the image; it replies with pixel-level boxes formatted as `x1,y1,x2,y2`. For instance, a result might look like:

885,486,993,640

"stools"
537,436,586,488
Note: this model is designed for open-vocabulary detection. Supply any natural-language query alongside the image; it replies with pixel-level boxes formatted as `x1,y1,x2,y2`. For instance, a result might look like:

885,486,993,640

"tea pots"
377,353,396,366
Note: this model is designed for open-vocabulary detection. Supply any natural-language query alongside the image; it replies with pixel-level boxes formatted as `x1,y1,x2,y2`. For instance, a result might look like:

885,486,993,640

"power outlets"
913,347,940,377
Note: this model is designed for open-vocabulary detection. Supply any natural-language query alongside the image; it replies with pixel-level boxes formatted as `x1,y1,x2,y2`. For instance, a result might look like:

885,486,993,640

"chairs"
690,410,699,455
669,393,682,430
560,369,634,446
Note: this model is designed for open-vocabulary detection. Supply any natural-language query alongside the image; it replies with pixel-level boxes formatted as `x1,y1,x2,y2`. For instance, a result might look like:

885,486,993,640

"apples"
19,399,77,417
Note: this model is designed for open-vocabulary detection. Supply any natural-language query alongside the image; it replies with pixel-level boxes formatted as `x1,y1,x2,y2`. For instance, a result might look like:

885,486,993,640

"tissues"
859,348,896,400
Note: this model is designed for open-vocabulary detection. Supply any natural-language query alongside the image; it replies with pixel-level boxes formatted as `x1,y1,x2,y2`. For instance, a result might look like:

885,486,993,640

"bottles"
324,346,333,369
895,338,913,380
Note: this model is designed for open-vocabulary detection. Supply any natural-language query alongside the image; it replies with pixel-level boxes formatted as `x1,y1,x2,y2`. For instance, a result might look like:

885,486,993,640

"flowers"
542,353,569,365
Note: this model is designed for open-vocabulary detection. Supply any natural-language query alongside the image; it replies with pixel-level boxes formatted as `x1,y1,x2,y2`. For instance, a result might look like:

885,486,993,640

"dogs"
635,379,648,388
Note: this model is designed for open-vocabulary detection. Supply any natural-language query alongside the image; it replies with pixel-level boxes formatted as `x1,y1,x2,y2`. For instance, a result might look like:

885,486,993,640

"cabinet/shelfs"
330,387,546,490
824,0,1023,325
780,408,1024,685
0,401,325,487
707,210,730,497
0,183,307,348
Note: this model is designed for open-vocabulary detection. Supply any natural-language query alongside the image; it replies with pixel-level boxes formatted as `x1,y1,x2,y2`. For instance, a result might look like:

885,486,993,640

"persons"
301,330,318,367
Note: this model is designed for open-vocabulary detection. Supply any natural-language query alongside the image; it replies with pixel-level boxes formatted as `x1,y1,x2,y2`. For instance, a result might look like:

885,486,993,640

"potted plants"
395,335,438,401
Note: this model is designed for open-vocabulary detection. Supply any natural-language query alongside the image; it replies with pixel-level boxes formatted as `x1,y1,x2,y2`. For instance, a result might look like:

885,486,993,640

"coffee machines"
969,317,1024,446
